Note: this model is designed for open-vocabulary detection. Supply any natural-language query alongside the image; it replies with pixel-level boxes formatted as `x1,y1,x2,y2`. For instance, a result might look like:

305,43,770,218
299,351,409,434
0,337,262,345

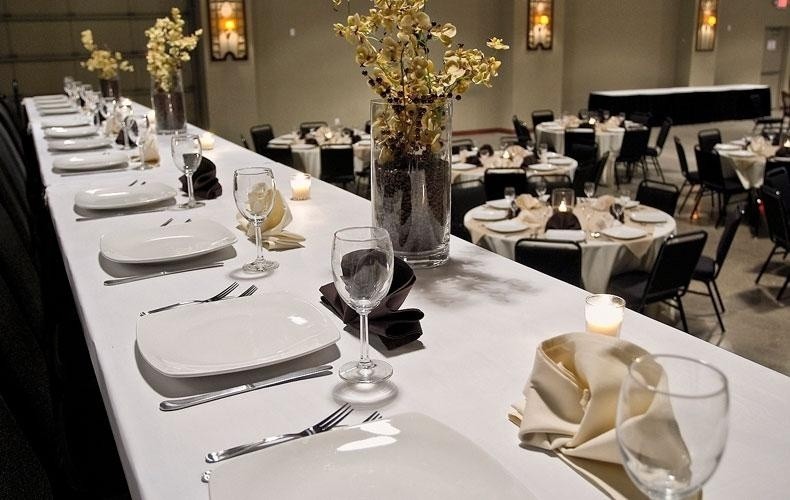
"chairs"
1,79,84,500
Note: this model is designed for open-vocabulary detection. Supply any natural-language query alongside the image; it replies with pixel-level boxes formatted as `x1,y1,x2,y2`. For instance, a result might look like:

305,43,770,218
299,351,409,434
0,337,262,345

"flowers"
329,2,510,253
80,27,135,83
142,7,205,106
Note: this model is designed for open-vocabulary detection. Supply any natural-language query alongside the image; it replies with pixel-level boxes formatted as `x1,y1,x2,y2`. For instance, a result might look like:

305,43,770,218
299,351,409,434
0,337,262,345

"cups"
290,173,311,202
584,294,626,336
621,352,729,497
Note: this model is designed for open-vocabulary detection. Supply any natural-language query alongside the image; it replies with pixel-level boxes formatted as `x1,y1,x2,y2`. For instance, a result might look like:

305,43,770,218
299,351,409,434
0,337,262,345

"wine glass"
502,183,638,220
56,77,204,213
550,110,626,122
329,226,396,385
234,166,279,276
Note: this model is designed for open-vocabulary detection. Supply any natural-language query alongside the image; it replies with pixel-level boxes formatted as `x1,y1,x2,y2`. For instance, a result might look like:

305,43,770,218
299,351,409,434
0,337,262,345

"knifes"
104,262,224,287
159,364,334,413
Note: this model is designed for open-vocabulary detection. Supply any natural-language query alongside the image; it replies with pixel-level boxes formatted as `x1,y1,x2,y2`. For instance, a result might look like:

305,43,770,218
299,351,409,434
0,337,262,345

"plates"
136,291,343,385
479,200,668,241
449,149,569,172
211,413,538,498
710,137,755,158
31,93,235,284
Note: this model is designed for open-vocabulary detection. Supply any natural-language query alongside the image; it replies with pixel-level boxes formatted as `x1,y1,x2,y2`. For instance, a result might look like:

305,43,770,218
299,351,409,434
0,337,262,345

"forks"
139,281,258,319
203,402,387,480
